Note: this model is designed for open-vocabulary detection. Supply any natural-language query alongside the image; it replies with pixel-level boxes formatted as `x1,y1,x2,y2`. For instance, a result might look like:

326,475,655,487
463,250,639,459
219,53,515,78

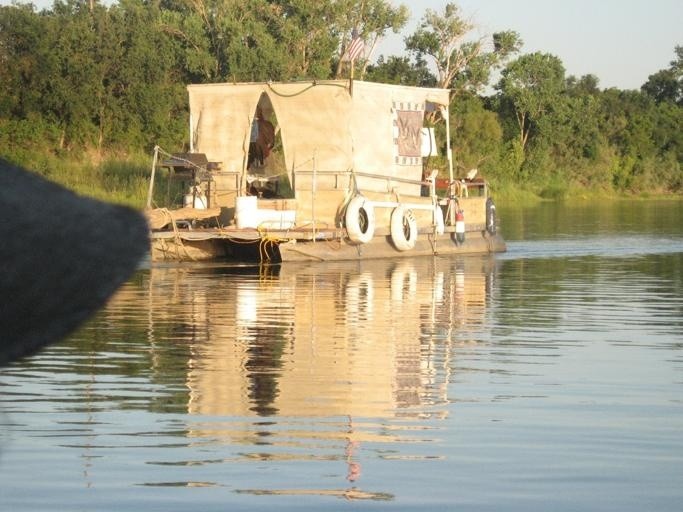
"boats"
136,74,508,262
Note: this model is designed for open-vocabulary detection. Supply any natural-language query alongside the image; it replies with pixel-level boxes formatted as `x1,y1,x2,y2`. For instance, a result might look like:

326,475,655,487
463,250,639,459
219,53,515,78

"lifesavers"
456,212,465,241
485,198,496,235
390,206,418,251
436,206,444,234
345,196,376,244
